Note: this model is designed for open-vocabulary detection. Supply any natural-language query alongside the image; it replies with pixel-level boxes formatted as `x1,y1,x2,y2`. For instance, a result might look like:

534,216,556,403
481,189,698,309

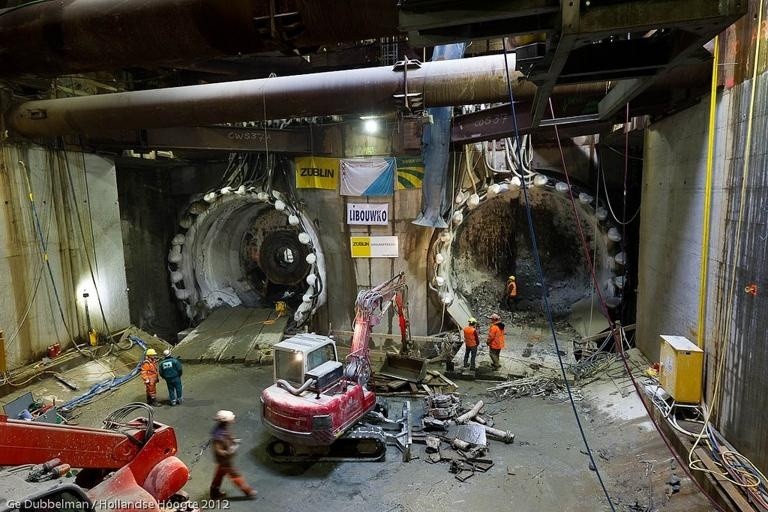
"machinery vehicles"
251,269,430,473
0,398,206,512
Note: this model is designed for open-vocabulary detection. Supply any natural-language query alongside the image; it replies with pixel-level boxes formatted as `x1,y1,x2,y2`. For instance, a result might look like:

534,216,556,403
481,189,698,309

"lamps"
83,289,91,331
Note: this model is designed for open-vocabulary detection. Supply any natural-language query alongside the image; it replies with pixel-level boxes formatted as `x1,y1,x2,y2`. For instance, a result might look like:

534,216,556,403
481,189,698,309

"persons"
506,275,517,311
159,349,185,407
486,313,505,371
209,409,259,500
462,317,480,371
140,348,162,407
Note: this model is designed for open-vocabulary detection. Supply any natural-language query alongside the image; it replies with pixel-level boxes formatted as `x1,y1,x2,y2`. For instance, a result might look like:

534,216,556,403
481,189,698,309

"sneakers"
463,361,501,371
208,487,258,499
147,398,184,406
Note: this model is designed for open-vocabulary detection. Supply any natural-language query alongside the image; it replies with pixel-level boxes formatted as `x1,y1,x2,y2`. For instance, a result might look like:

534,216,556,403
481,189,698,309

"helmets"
509,275,515,280
163,349,171,357
213,410,236,422
146,348,157,356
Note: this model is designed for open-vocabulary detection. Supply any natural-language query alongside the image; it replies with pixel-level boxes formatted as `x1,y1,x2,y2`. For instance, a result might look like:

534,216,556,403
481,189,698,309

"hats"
488,313,500,320
468,317,478,323
